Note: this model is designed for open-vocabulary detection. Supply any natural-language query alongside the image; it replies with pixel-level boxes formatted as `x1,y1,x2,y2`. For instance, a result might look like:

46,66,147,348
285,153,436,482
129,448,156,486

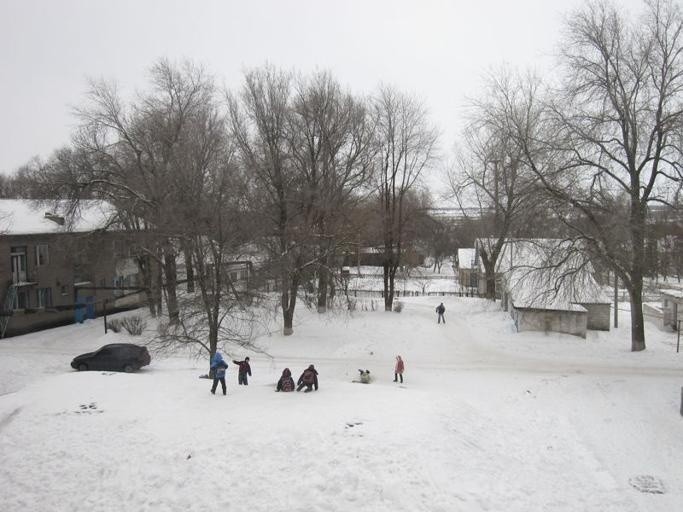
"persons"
393,355,404,383
297,364,319,393
352,369,372,384
209,352,228,395
232,356,252,385
436,302,446,324
275,368,295,393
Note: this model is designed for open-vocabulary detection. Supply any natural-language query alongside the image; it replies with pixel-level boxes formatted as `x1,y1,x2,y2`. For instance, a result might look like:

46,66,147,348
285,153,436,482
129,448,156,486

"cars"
71,344,149,372
